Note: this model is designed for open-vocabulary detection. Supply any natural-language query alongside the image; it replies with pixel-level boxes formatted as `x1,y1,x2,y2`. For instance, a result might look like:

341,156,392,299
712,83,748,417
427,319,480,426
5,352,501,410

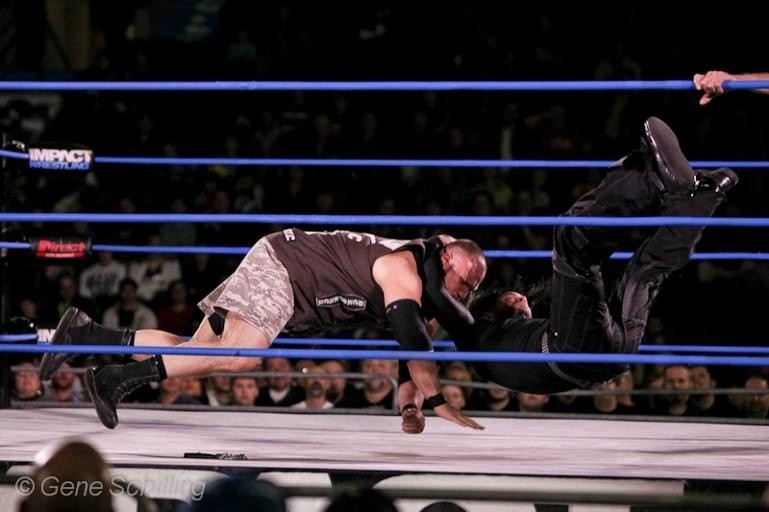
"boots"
38,306,136,382
84,354,168,430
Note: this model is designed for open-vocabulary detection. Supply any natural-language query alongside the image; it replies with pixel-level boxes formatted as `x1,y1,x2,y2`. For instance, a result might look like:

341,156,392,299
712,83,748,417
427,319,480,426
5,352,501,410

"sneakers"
692,167,739,192
644,117,698,201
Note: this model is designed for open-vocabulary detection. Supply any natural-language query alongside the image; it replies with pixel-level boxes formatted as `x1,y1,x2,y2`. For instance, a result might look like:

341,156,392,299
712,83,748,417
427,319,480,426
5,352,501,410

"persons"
16,440,468,512
0,40,769,433
